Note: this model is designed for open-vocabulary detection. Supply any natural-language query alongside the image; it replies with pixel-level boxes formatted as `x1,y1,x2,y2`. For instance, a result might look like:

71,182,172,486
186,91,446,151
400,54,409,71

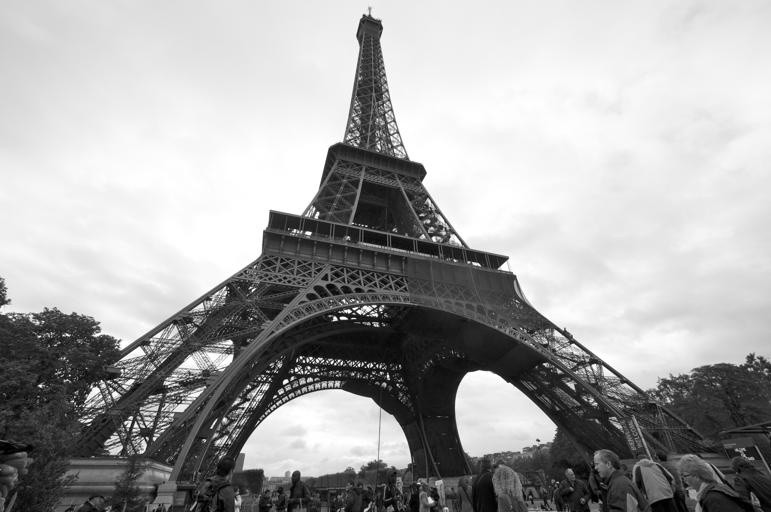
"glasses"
681,473,692,480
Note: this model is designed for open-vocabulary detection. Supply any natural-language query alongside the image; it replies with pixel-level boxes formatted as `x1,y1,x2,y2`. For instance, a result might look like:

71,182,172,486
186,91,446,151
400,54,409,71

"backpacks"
189,478,230,512
